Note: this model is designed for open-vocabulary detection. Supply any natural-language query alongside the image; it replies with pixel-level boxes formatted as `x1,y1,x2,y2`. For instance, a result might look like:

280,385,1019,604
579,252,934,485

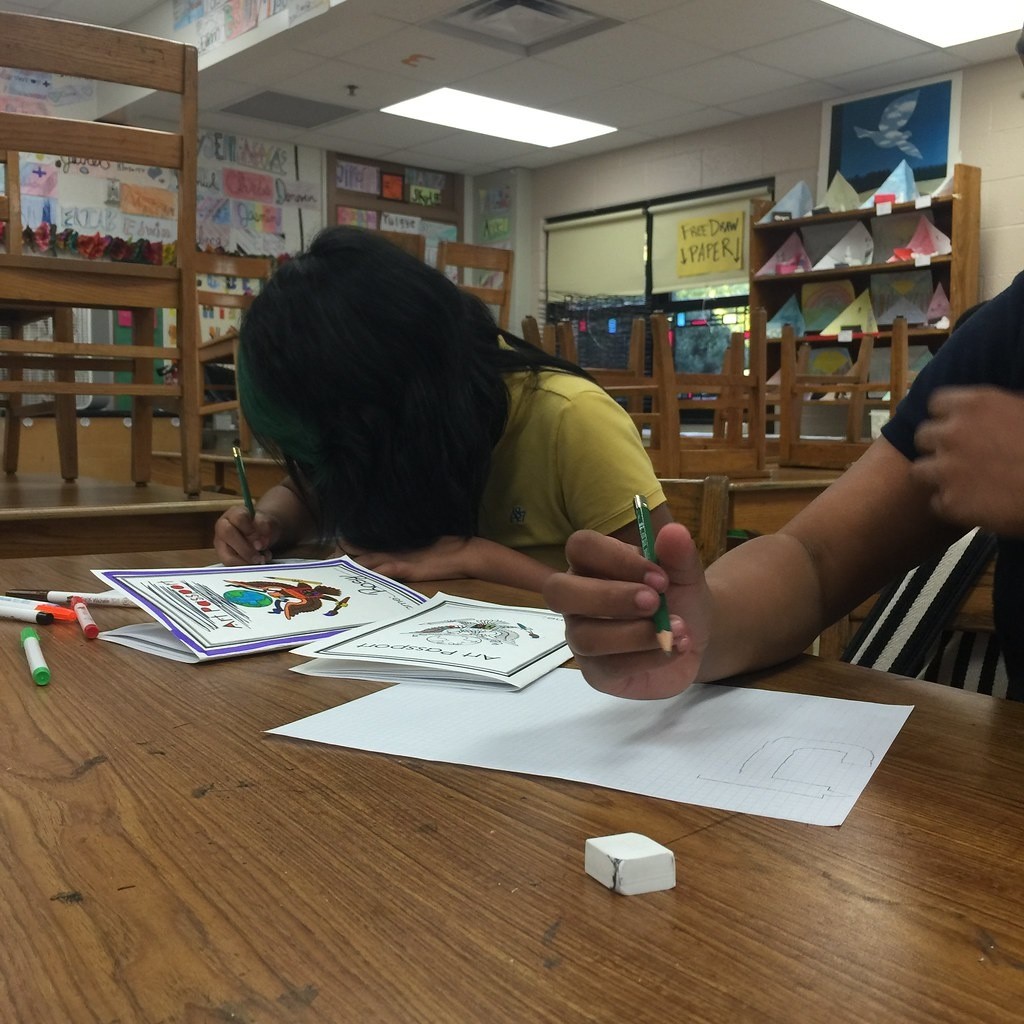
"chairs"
0,9,1024,699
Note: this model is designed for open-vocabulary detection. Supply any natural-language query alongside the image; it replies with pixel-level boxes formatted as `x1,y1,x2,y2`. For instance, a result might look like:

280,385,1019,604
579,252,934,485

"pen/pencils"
233,447,265,564
634,491,674,659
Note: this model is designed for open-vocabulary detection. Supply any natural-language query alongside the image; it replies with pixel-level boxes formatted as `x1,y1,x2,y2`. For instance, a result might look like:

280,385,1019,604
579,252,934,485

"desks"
657,461,849,659
0,471,261,559
0,555,1024,1024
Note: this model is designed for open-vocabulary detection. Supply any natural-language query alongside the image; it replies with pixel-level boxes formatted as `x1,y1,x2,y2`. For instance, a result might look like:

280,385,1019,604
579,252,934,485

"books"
89,553,431,665
287,590,575,692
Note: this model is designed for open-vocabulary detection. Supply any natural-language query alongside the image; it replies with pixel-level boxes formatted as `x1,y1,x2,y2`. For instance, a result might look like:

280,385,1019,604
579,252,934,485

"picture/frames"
815,70,963,208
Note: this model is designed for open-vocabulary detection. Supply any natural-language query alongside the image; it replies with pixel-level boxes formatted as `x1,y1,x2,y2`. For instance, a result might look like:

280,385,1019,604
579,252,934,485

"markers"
0,596,77,625
70,596,99,640
5,590,139,607
19,628,50,686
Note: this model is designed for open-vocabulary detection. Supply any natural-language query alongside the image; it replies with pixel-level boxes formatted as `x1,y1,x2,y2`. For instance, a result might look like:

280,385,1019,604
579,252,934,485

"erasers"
586,832,679,895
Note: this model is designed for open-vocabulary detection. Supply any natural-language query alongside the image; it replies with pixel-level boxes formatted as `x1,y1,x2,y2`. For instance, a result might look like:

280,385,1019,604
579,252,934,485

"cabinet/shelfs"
749,164,980,439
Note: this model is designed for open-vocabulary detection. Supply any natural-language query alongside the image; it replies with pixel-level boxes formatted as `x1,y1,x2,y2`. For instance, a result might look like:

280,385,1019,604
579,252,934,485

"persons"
214,223,674,591
543,270,1024,704
